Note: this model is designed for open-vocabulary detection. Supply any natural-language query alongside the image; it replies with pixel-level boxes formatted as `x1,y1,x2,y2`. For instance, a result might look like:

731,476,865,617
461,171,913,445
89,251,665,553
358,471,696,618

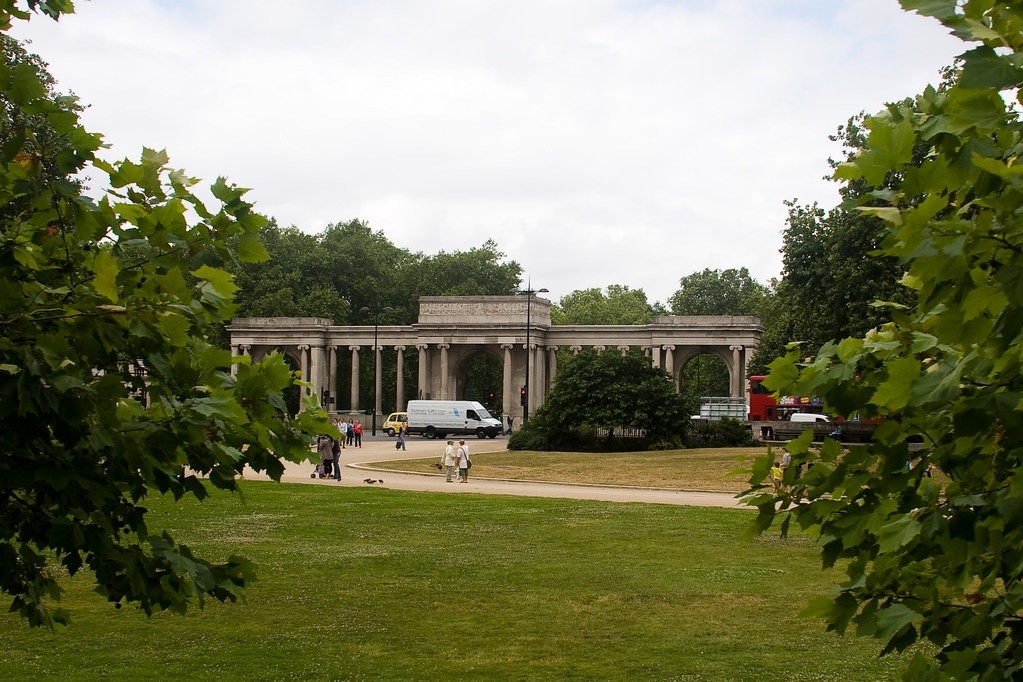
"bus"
748,376,886,423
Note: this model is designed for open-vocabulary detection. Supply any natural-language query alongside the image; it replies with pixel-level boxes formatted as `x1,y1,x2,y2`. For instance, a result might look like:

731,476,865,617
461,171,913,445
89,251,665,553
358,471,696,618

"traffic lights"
521,388,527,406
323,390,330,403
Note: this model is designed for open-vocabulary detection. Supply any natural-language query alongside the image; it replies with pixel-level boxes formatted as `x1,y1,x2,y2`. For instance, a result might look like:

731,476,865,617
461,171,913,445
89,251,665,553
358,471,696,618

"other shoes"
460,481,468,483
446,480,453,482
332,477,336,479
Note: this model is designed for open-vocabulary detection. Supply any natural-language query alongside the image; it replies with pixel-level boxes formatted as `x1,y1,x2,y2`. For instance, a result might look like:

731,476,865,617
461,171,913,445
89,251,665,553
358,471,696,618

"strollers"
310,459,334,478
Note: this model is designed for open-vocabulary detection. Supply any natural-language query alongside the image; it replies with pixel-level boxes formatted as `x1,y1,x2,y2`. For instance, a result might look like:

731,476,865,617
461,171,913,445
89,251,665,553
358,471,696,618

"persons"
317,434,341,481
352,419,363,448
505,413,513,435
771,462,784,493
782,448,791,472
332,418,354,448
441,440,457,482
397,422,407,451
905,457,914,471
456,439,469,483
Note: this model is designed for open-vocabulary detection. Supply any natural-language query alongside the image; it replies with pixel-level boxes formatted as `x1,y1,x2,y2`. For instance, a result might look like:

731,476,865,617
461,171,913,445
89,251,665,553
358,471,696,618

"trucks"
790,413,831,423
406,401,502,439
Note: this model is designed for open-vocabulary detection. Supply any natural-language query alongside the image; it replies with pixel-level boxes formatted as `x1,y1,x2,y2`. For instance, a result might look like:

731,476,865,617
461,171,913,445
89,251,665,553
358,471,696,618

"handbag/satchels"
317,464,326,475
396,438,401,449
467,460,471,469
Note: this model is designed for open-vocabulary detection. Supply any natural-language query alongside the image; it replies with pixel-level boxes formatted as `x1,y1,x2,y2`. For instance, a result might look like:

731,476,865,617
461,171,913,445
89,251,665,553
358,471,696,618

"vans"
382,412,427,437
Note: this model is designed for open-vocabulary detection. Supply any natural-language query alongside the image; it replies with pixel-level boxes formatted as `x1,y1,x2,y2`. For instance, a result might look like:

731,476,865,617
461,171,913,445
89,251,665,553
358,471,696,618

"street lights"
361,307,393,436
508,276,549,424
